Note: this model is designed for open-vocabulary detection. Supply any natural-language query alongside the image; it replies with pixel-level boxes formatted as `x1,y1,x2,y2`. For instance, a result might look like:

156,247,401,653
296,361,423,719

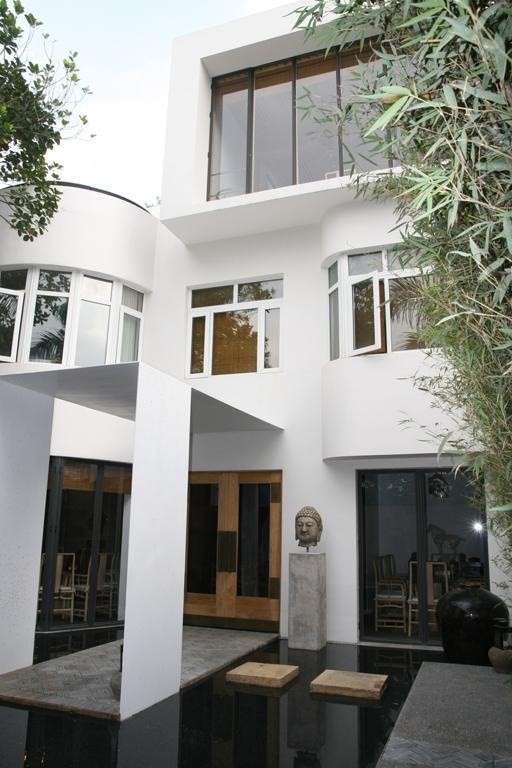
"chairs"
372,555,448,636
40,552,119,623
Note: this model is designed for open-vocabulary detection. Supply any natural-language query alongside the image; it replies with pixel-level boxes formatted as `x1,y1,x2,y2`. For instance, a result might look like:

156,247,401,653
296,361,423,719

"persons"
291,503,324,548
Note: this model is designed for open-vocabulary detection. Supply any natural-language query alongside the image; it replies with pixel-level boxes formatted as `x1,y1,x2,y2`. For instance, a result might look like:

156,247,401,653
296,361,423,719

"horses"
427,524,466,562
